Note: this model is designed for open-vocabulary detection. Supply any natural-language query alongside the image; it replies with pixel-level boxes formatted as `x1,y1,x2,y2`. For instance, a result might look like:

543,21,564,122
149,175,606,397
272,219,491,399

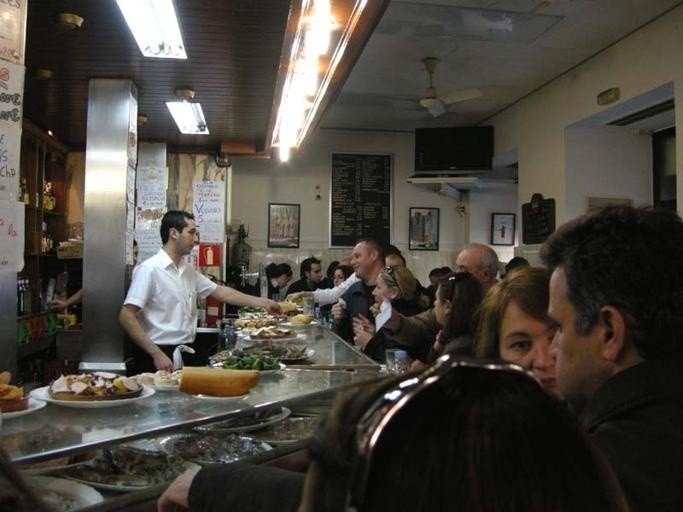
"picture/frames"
409,208,439,251
586,196,635,214
267,203,300,248
490,212,516,245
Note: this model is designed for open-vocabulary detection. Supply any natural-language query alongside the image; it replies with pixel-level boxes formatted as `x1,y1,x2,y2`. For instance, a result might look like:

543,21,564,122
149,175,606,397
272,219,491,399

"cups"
384,349,406,374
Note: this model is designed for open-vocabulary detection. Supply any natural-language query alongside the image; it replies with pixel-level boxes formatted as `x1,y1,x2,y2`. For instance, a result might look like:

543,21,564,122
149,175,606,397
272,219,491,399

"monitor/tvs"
415,126,494,175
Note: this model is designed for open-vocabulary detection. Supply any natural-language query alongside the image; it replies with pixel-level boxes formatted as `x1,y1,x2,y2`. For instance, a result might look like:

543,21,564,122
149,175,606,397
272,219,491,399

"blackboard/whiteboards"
522,193,555,244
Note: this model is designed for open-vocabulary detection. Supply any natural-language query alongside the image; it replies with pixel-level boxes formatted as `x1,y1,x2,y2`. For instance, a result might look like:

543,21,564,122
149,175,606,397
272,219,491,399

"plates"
135,370,178,392
29,384,155,408
67,449,192,491
0,399,46,419
194,391,247,403
193,406,292,434
5,426,100,474
209,293,318,375
3,474,103,511
160,432,273,465
247,415,319,446
113,421,171,453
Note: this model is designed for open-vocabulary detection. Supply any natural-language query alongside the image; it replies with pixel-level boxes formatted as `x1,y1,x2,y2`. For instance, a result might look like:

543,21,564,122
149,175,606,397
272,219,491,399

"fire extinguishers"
205,273,226,326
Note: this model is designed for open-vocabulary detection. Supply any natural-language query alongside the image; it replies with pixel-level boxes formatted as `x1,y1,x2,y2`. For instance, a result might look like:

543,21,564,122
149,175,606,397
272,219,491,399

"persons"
132,238,140,267
156,199,682,511
118,209,285,378
45,288,82,312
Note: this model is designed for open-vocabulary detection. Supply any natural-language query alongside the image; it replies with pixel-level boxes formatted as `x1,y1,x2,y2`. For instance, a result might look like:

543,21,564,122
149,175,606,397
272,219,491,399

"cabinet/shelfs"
15,118,70,384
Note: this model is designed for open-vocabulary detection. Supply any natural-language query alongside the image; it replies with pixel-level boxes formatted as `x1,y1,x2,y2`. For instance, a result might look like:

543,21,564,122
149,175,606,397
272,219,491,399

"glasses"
385,266,397,285
346,354,542,512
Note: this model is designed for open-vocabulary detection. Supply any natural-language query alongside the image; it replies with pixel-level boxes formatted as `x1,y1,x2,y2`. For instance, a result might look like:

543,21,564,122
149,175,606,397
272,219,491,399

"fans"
387,57,483,118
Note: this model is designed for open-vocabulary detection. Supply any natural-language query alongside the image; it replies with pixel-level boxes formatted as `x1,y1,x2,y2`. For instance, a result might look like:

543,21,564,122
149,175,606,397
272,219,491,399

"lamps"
165,101,210,136
263,0,390,165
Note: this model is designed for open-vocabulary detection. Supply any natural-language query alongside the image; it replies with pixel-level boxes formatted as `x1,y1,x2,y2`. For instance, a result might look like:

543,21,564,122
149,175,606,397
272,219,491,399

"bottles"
15,276,33,317
18,177,56,210
196,299,207,327
